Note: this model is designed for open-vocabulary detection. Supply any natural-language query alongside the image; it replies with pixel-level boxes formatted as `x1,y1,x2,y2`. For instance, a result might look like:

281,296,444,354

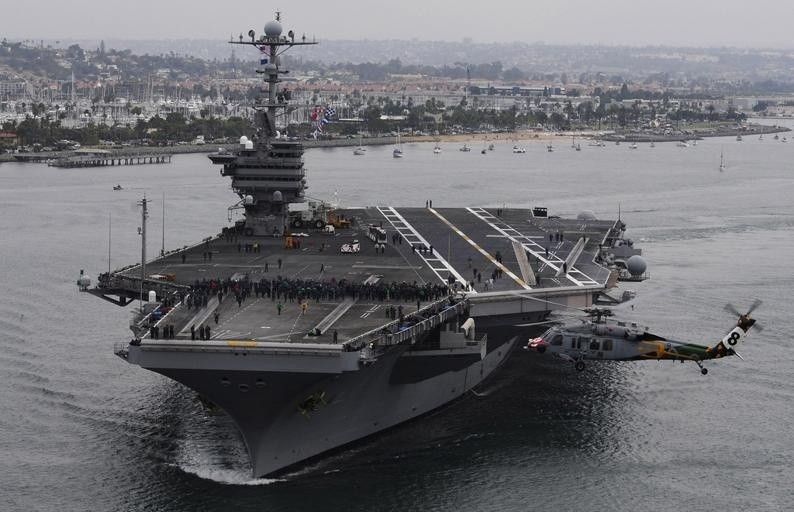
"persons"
473,267,503,291
563,262,567,273
546,247,549,255
526,251,530,261
495,252,502,263
149,200,474,344
535,271,541,286
549,232,563,242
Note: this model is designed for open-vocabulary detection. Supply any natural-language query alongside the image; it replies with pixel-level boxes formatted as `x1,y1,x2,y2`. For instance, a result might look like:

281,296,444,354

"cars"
60,140,80,150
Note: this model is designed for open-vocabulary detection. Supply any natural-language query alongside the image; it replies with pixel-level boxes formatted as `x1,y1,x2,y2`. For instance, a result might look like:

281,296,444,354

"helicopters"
513,292,763,375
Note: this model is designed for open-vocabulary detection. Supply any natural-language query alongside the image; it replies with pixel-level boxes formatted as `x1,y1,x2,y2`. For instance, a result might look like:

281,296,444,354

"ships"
78,11,650,478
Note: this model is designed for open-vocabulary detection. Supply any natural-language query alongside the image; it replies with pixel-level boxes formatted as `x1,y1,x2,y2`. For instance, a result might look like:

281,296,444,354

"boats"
113,186,123,190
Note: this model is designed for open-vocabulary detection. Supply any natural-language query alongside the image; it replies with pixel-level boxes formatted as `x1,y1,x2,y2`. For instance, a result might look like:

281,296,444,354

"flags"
310,106,336,141
